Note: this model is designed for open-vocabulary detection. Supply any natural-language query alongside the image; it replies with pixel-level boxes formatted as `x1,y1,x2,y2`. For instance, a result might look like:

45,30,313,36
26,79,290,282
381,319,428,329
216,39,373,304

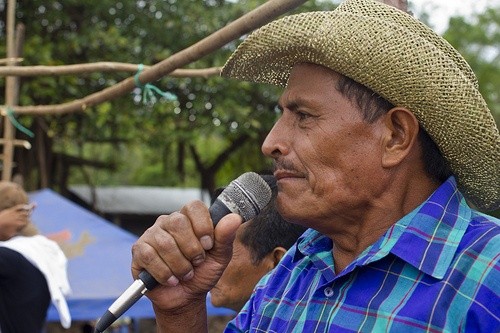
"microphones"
95,172,272,332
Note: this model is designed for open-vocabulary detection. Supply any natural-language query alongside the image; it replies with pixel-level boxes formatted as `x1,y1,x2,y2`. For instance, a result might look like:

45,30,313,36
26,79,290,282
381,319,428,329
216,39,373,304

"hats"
219,1,500,212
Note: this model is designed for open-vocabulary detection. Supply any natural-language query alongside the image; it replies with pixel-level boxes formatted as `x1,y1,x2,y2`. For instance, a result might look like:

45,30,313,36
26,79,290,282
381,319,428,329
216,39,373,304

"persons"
0,181,73,333
208,169,310,314
130,0,500,333
